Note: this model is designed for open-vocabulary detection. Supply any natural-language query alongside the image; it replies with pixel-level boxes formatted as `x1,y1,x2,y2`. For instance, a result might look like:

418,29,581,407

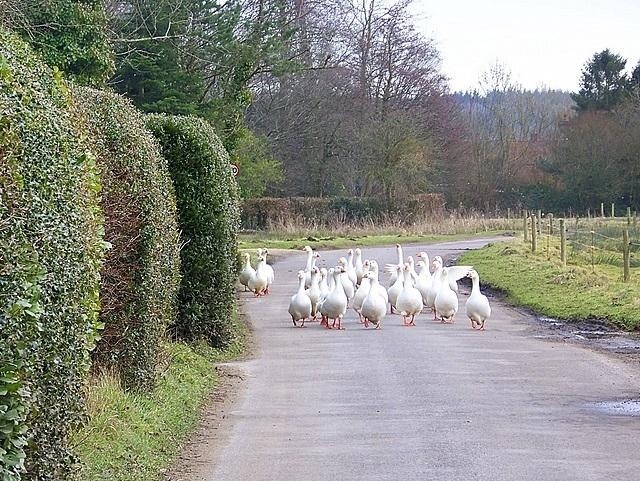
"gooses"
239,248,275,299
288,243,492,331
